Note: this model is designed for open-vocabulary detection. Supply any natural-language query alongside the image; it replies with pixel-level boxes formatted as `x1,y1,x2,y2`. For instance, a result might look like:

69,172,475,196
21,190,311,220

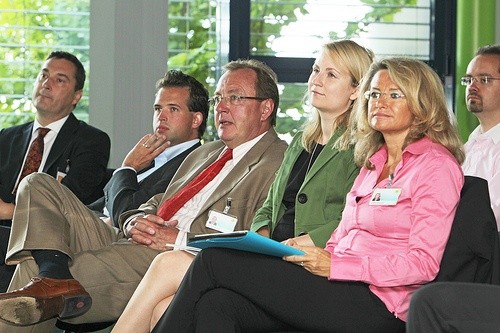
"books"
187,230,304,258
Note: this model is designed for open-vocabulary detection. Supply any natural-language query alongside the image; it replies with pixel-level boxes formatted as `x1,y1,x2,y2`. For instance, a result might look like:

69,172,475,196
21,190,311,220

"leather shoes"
0,273,94,326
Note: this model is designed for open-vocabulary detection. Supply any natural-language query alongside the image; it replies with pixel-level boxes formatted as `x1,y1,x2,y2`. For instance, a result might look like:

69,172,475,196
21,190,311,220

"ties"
137,160,154,175
154,149,233,221
13,127,51,195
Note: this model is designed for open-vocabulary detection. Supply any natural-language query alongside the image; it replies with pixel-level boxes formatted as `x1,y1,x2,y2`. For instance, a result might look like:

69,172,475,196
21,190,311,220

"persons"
152,57,466,333
406,282,500,333
110,40,375,333
0,50,210,293
209,216,217,225
372,193,380,201
460,44,500,232
0,60,289,333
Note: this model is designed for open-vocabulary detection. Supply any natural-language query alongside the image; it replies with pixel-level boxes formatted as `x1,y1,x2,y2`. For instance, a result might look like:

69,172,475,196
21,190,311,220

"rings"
301,261,303,266
143,143,148,148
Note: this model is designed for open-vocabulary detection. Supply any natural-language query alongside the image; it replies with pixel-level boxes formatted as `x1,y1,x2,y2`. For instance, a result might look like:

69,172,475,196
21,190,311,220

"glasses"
207,93,269,108
362,90,405,104
461,74,500,87
